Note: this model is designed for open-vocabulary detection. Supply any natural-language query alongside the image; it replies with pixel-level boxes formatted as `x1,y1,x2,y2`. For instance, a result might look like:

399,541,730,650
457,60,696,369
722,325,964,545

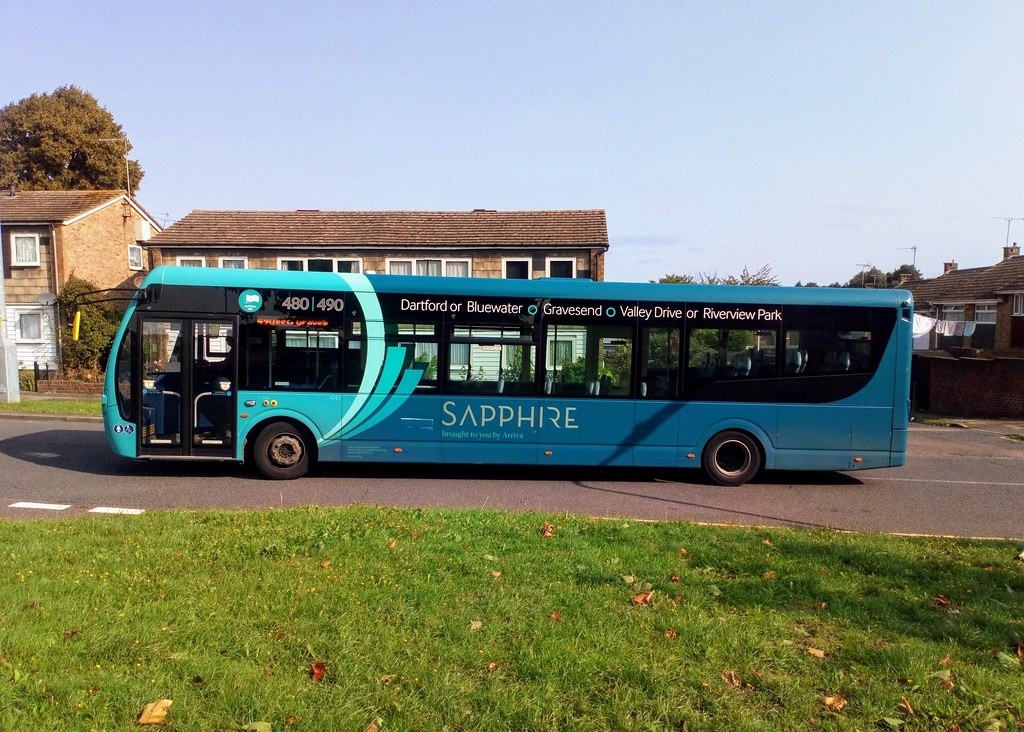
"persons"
205,329,236,377
153,360,163,373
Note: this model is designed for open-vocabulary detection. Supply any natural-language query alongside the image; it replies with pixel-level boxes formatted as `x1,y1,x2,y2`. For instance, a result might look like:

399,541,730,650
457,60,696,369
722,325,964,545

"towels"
913,313,976,338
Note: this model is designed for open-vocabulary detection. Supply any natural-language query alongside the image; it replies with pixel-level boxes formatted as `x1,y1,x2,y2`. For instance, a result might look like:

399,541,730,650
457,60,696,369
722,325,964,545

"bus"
72,265,916,488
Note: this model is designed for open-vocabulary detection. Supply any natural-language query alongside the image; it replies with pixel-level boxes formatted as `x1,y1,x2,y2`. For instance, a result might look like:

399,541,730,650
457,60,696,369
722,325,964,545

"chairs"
700,346,854,377
495,369,612,397
